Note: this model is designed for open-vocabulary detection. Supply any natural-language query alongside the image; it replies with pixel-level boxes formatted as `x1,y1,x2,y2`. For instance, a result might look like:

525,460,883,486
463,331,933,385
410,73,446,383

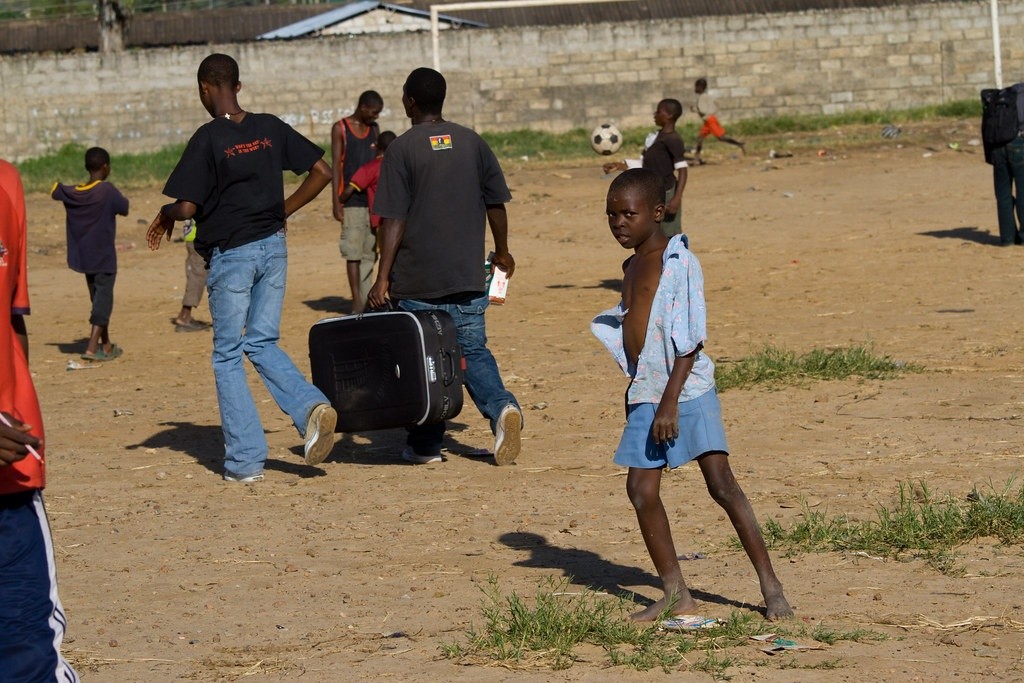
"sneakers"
402,447,442,464
304,405,337,466
494,405,522,466
224,470,263,483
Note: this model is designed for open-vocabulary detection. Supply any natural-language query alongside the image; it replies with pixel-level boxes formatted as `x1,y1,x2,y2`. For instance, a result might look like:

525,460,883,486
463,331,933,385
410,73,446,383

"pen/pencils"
0,413,44,464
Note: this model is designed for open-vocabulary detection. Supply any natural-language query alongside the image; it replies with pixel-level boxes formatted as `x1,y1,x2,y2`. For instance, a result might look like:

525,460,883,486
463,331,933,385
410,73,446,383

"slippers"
101,343,123,361
663,613,728,630
171,316,208,330
81,351,101,362
750,632,824,653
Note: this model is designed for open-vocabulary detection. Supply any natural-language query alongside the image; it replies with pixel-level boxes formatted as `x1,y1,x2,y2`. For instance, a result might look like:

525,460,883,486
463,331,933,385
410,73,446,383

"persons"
603,99,690,243
330,89,384,316
145,52,338,483
688,78,747,164
360,68,525,469
339,129,397,264
168,217,212,333
0,157,75,683
992,83,1024,246
587,169,796,622
50,147,131,361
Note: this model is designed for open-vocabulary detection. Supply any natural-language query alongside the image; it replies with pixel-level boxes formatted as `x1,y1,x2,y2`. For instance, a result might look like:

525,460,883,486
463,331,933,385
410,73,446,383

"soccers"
590,123,624,155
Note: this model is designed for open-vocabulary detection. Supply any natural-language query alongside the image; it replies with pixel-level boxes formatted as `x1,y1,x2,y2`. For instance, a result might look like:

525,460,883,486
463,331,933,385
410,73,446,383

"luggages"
312,299,464,434
